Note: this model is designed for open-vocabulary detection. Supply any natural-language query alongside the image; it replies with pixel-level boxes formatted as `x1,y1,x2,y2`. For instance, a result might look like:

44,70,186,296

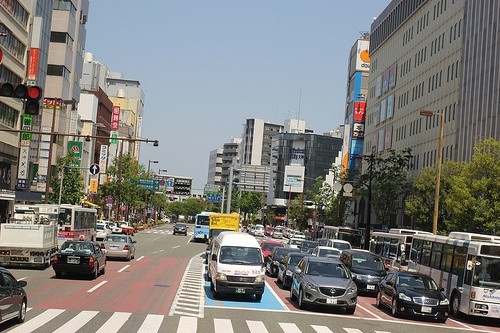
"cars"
173,224,189,236
52,240,108,279
377,271,450,324
96,220,136,240
261,240,315,289
0,267,27,326
243,224,304,240
287,237,327,253
101,234,136,261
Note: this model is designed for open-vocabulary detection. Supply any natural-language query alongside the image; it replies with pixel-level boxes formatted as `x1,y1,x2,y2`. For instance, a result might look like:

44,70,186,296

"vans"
208,230,265,300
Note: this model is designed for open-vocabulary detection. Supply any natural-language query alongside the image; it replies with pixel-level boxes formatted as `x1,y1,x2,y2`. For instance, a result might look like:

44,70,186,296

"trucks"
0,212,58,270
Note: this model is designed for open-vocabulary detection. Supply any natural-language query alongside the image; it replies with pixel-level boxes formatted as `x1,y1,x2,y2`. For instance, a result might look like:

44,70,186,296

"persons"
221,249,234,261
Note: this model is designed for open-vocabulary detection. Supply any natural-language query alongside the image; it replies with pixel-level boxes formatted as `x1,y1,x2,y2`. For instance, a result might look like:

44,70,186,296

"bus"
323,224,500,321
194,211,241,245
14,204,98,248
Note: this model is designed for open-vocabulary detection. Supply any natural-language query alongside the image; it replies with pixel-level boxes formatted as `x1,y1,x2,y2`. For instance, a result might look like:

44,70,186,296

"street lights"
146,160,167,175
420,110,444,233
273,153,374,250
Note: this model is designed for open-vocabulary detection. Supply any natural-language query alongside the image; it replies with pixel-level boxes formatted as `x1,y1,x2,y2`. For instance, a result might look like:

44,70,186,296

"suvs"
312,239,389,297
291,257,357,314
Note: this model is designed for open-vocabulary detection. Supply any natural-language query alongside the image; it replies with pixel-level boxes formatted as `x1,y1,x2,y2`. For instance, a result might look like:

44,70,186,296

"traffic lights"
0,82,42,115
